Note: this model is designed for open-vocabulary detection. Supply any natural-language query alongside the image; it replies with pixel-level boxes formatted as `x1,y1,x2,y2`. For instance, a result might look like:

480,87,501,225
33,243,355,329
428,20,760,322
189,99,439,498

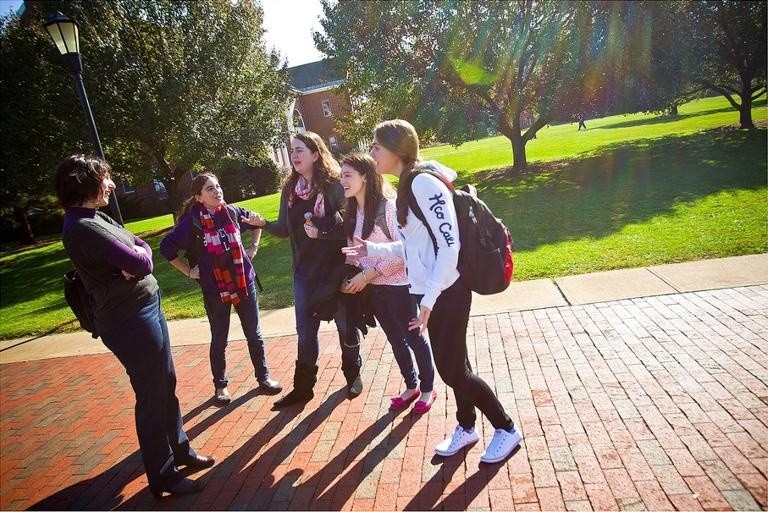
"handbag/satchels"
62,266,102,344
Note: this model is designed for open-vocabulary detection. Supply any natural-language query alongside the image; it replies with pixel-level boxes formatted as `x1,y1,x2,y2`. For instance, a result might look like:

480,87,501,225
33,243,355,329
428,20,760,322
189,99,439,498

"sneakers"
434,425,481,457
480,427,524,464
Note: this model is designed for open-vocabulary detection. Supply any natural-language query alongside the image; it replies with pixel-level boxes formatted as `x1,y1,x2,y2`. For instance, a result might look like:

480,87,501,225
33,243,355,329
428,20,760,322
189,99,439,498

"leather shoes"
412,390,438,414
389,388,421,410
214,387,231,404
258,378,282,394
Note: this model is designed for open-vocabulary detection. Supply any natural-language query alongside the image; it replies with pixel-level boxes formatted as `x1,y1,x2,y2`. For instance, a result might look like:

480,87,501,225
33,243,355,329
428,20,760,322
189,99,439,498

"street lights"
42,12,126,230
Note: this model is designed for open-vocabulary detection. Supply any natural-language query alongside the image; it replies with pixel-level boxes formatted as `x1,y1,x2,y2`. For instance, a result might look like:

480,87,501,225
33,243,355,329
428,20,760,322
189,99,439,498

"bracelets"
373,266,384,277
189,271,190,278
361,269,372,285
249,242,259,248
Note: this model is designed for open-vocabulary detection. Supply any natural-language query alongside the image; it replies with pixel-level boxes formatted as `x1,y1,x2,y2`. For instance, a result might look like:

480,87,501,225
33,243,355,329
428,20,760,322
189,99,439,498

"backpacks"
406,165,515,296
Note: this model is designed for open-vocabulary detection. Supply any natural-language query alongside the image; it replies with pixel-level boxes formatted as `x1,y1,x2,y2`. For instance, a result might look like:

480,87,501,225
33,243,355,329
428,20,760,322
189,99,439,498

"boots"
341,357,364,398
273,360,318,409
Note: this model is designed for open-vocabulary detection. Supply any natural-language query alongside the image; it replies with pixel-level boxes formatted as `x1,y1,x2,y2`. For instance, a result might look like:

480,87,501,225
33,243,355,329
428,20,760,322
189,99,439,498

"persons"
241,131,363,406
340,154,436,415
576,111,587,131
160,173,283,406
368,119,523,465
53,153,215,497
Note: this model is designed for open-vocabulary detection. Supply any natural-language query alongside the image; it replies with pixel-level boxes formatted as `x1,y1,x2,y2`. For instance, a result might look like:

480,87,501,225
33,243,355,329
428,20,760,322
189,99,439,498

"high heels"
148,475,208,498
173,449,216,469
